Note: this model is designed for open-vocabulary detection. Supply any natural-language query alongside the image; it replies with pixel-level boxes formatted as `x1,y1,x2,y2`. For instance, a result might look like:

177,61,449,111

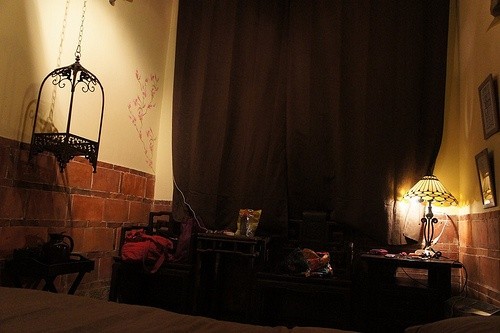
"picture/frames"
475,148,496,209
478,73,500,140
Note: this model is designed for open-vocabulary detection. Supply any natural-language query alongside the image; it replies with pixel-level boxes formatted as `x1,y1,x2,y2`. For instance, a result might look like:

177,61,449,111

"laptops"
234,208,262,237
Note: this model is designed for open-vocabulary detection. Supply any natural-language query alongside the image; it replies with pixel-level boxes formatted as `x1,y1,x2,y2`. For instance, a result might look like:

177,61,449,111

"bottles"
240,211,249,237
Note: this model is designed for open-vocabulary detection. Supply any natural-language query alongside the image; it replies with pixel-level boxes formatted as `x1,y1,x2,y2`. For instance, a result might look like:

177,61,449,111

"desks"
360,253,463,333
22,253,95,294
191,232,271,314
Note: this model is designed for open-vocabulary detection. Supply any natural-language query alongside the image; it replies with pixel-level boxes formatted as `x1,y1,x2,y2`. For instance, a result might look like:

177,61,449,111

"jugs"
40,231,74,263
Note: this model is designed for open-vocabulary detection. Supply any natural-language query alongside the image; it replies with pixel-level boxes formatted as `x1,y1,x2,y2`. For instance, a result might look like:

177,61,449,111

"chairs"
109,211,355,329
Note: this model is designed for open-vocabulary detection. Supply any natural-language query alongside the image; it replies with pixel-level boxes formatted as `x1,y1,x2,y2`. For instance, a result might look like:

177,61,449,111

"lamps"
403,175,458,255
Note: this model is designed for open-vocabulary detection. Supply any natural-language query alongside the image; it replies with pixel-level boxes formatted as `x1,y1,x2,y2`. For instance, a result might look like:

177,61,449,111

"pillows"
406,315,500,333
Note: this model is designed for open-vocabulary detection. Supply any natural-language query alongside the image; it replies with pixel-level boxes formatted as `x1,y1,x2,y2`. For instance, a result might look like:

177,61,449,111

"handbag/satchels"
121,228,174,274
285,248,309,274
304,248,334,279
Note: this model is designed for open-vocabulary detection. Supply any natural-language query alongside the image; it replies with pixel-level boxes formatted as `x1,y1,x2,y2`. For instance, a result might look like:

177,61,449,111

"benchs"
0,287,360,333
